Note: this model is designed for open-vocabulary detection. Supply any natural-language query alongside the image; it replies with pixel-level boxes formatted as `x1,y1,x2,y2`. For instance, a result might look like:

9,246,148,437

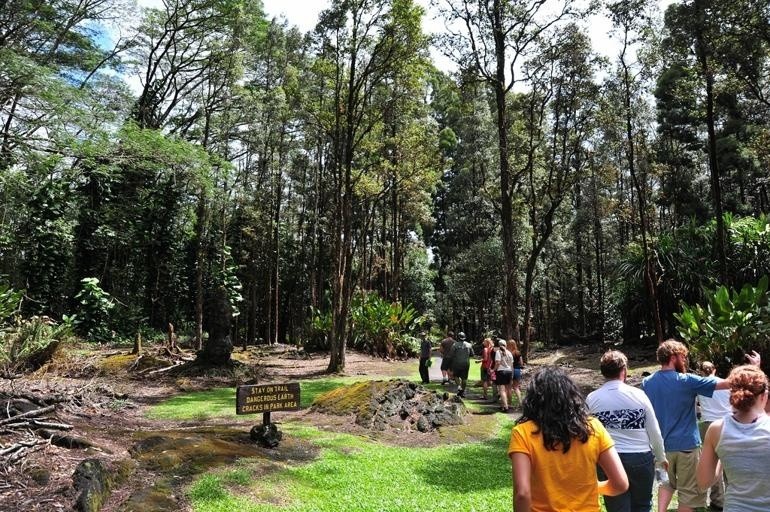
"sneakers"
458,387,465,398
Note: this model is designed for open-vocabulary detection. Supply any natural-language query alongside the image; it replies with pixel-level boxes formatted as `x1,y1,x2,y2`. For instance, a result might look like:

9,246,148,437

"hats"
458,332,466,340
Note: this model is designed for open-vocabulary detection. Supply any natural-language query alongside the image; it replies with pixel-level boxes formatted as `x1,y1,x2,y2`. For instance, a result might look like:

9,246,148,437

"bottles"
652,464,671,487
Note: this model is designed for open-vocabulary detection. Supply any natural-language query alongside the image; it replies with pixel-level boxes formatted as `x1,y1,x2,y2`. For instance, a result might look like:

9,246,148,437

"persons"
642,338,762,511
419,331,433,384
507,362,632,512
437,331,525,413
694,366,770,512
582,349,670,511
695,359,737,512
